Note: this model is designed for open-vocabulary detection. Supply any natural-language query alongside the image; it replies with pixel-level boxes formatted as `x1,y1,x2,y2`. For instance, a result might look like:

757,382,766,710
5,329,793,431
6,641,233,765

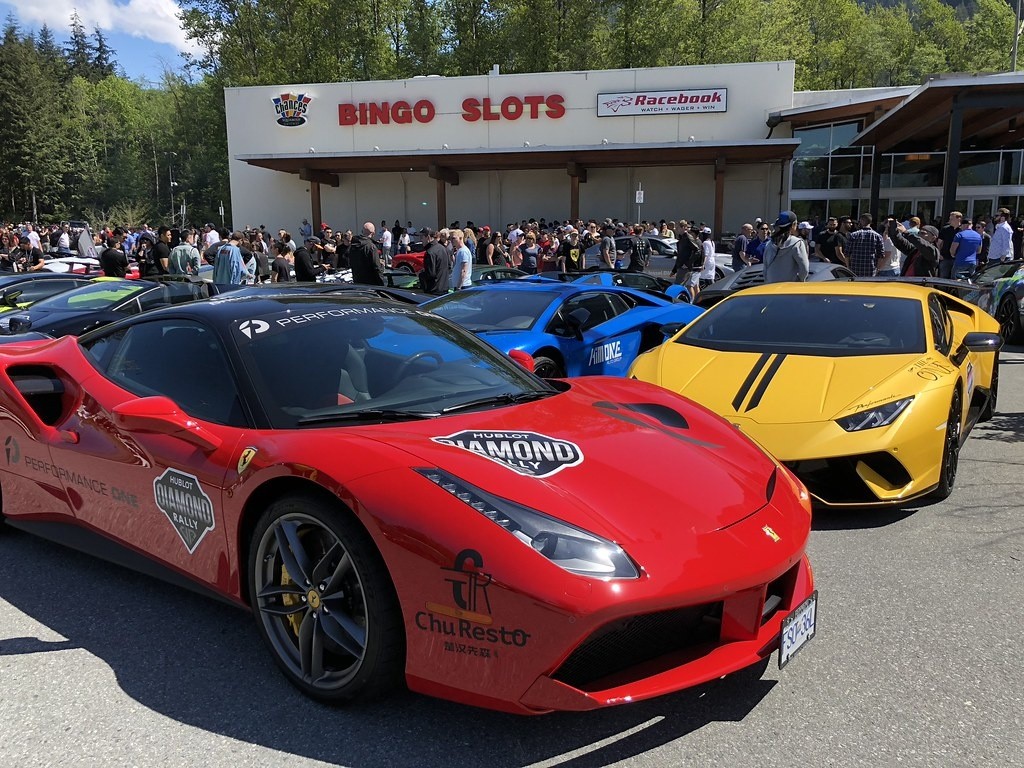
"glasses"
278,232,281,235
995,214,1000,217
748,229,754,231
845,221,851,224
591,226,595,228
3,238,6,240
497,236,501,237
327,231,331,233
760,228,768,230
976,227,980,229
122,235,128,239
335,235,339,236
65,226,70,229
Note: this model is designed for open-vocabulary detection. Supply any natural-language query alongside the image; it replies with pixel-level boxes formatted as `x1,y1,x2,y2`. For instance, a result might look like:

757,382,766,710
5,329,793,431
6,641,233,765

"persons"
397,229,410,254
629,218,716,304
348,221,385,286
135,232,156,278
624,225,651,273
271,229,296,282
99,223,156,254
50,222,72,253
271,242,291,282
99,238,129,277
298,219,311,246
439,227,472,292
317,223,353,268
167,224,219,264
415,226,449,296
450,218,628,275
375,219,417,258
0,221,50,272
762,211,809,284
203,225,271,285
153,226,172,274
169,229,200,275
292,236,330,282
732,208,1024,277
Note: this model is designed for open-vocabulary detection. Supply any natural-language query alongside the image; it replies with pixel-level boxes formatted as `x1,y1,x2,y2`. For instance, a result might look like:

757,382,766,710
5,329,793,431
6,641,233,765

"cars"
582,236,735,281
631,282,1004,517
1,280,208,341
1,264,88,309
391,251,427,274
972,259,1024,343
695,262,857,312
400,264,529,295
416,281,707,378
530,271,690,303
1,294,818,718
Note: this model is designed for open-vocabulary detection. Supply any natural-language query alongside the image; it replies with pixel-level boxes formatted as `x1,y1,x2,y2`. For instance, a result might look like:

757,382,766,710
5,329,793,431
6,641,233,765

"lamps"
687,136,695,143
309,148,314,153
373,146,378,151
523,141,529,147
442,144,448,149
601,139,607,144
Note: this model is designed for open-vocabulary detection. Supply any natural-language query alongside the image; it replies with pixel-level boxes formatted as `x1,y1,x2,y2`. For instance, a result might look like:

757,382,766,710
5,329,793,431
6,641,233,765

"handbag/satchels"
407,245,411,252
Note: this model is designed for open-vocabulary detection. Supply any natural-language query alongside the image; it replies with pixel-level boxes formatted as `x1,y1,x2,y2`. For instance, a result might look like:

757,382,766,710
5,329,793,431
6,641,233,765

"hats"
660,219,665,222
538,229,548,234
775,211,797,227
700,222,705,225
961,218,972,224
755,218,762,222
307,236,323,249
420,226,431,233
302,219,307,222
699,227,711,234
483,226,490,231
921,225,938,237
798,221,814,229
321,223,328,229
617,223,624,226
909,216,920,224
570,229,578,236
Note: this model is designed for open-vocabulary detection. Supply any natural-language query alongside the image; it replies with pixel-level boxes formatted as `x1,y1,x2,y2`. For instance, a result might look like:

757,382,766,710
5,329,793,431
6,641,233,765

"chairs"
303,342,371,405
